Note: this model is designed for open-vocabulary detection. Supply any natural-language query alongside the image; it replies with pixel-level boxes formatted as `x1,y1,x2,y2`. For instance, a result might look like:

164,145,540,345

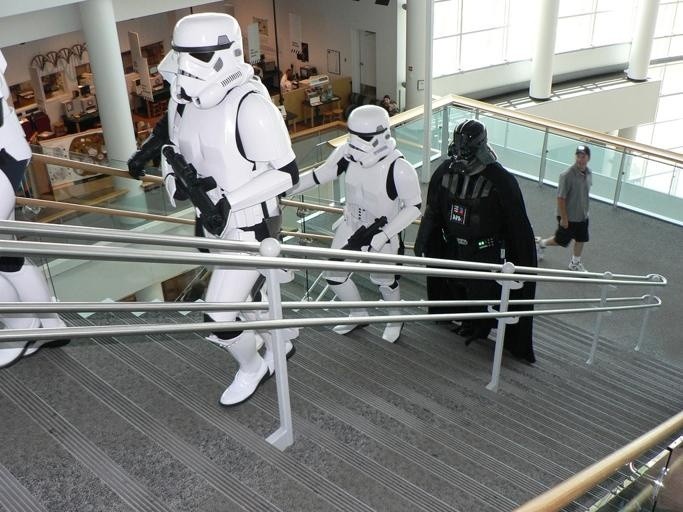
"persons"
536,145,593,274
381,95,398,114
160,12,296,407
281,105,422,344
279,69,297,92
0,51,72,370
416,119,537,364
126,50,270,351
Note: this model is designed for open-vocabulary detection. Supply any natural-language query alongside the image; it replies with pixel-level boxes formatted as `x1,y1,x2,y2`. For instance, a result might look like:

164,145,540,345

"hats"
575,145,590,156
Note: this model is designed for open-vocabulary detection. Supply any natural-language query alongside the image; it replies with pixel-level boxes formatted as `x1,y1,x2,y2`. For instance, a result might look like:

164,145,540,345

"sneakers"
534,236,546,262
568,259,587,272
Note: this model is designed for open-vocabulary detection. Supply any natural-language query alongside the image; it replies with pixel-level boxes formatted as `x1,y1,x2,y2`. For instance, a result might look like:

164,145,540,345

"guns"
162,147,222,227
328,216,387,260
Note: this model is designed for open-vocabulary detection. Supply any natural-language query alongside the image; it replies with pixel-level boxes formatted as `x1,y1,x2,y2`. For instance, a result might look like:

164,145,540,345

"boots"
248,288,295,376
213,315,270,407
378,279,405,343
1,258,70,370
329,276,370,334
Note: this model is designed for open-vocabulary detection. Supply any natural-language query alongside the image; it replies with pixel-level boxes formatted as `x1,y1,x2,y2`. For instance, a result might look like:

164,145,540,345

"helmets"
169,12,255,109
447,118,498,176
344,104,397,169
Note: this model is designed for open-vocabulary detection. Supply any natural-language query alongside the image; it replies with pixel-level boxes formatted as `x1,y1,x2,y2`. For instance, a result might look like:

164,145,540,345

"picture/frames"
417,79,424,90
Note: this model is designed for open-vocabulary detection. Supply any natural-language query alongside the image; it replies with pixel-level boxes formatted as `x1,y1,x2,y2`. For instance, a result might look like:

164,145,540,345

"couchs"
345,93,396,120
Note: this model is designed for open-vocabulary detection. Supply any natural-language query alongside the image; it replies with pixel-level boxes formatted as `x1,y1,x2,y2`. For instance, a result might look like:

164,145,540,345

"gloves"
128,143,151,180
197,195,230,237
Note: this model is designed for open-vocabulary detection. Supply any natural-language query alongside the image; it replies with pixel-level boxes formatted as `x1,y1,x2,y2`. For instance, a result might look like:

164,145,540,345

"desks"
301,95,342,128
60,108,99,133
129,88,169,118
286,111,299,134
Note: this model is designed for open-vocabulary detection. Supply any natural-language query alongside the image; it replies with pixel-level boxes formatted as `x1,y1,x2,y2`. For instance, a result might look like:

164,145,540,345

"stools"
321,109,344,125
151,98,168,118
